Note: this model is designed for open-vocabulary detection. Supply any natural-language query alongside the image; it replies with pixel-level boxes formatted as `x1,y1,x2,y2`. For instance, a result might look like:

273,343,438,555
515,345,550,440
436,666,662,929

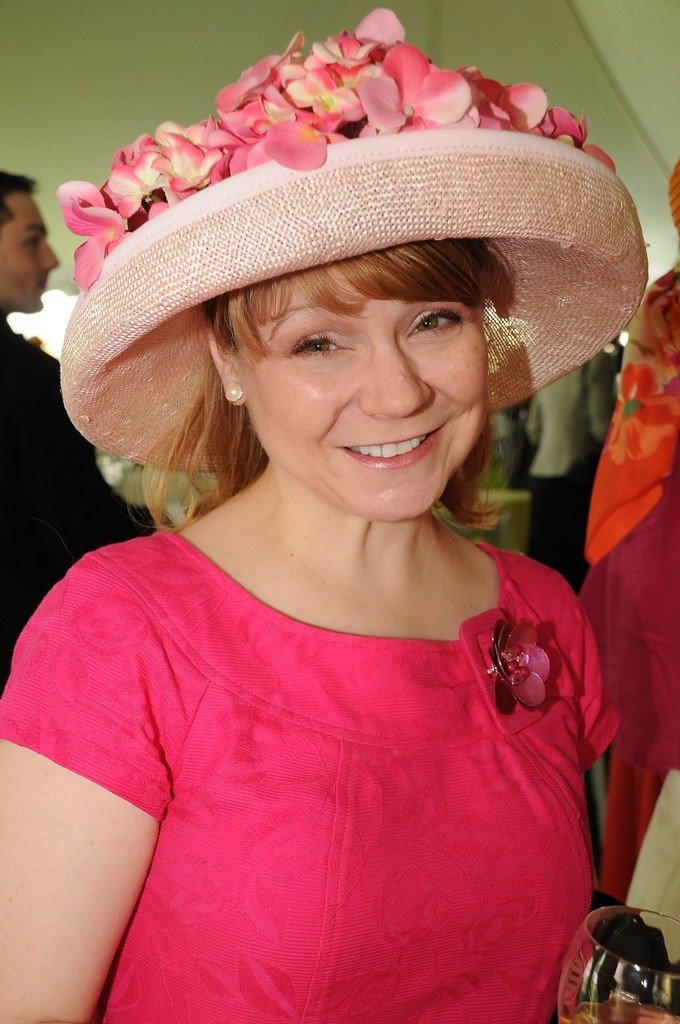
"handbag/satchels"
507,428,533,487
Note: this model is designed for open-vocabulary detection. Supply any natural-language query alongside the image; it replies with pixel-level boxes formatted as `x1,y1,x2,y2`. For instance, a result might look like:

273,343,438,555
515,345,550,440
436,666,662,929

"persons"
0,8,680,1024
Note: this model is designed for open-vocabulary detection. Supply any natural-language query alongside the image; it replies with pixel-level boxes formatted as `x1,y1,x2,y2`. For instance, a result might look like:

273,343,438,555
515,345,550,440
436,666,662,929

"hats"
57,6,649,470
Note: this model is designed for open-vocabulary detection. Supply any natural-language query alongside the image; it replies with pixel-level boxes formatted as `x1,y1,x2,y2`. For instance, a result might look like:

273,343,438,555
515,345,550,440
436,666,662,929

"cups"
557,905,680,1024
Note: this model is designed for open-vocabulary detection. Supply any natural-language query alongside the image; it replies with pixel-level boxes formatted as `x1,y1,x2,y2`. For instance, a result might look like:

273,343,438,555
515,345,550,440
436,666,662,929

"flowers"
486,614,553,714
54,9,615,293
